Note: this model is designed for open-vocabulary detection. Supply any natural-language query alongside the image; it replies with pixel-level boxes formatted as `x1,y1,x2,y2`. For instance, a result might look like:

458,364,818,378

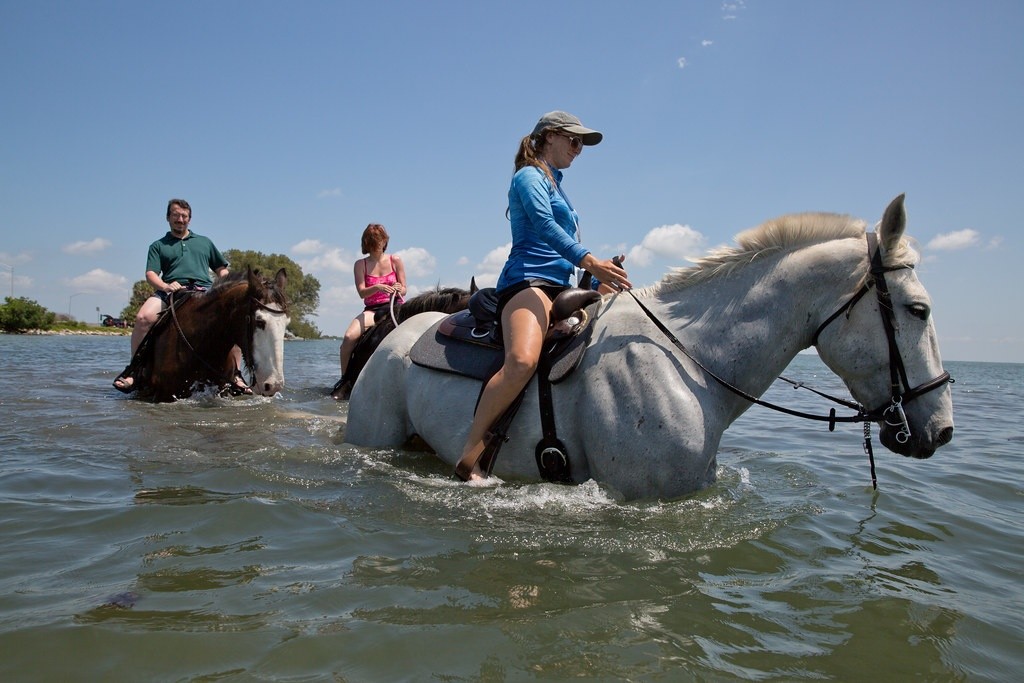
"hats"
532,111,603,146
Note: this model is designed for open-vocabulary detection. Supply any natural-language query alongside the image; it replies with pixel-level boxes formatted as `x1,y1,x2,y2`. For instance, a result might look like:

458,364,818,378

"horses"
138,264,292,403
342,192,955,504
347,276,480,397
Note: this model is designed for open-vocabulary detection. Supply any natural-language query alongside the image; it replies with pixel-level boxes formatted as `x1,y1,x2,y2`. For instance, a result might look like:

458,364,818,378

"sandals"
112,377,132,391
234,379,253,395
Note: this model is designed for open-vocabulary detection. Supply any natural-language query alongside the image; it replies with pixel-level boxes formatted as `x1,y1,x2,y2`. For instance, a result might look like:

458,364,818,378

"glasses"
554,132,584,156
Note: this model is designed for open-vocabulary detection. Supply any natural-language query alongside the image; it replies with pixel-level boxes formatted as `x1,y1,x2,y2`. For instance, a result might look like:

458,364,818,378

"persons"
456,109,635,478
111,198,253,395
340,223,407,377
